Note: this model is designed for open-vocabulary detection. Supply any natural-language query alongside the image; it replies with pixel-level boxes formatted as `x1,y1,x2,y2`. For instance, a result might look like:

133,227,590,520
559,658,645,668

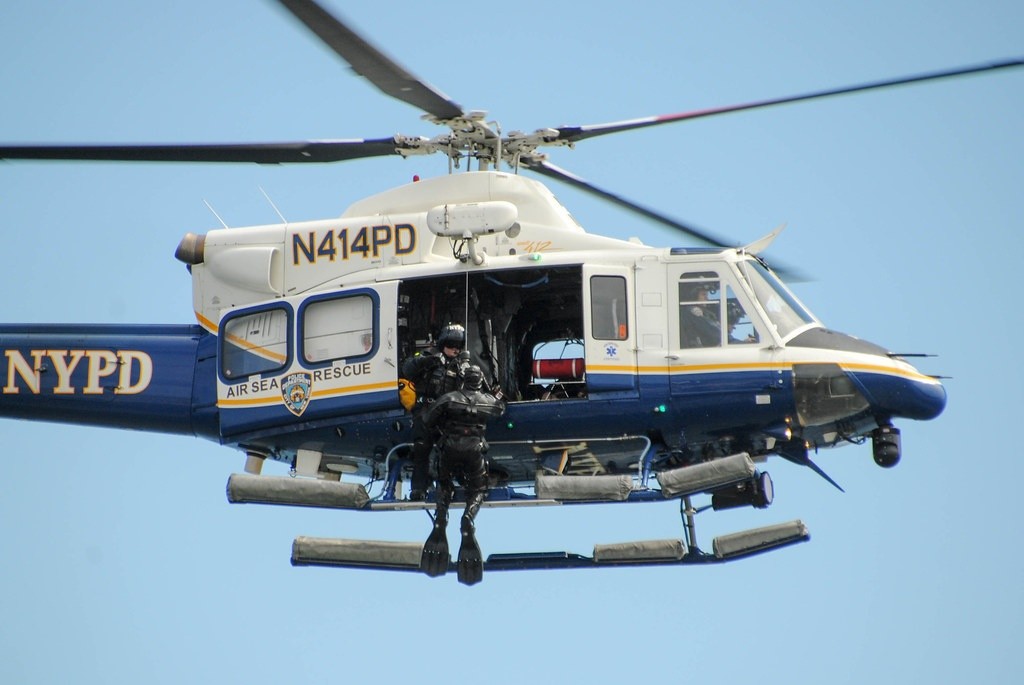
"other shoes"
410,489,428,500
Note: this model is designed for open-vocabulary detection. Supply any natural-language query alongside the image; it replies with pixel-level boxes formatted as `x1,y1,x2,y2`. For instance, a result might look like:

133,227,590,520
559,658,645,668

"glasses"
445,341,463,349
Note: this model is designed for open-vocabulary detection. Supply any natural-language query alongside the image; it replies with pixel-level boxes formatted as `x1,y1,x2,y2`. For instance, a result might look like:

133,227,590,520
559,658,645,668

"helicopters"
1,1,1023,573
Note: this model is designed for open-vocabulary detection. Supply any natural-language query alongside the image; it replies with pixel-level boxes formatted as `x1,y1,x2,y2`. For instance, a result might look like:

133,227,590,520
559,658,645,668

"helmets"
439,324,465,352
681,274,713,300
715,302,743,323
464,365,484,391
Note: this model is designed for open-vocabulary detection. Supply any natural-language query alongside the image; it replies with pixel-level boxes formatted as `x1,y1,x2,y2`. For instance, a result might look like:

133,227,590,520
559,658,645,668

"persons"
679,274,757,348
420,366,506,586
403,321,468,500
